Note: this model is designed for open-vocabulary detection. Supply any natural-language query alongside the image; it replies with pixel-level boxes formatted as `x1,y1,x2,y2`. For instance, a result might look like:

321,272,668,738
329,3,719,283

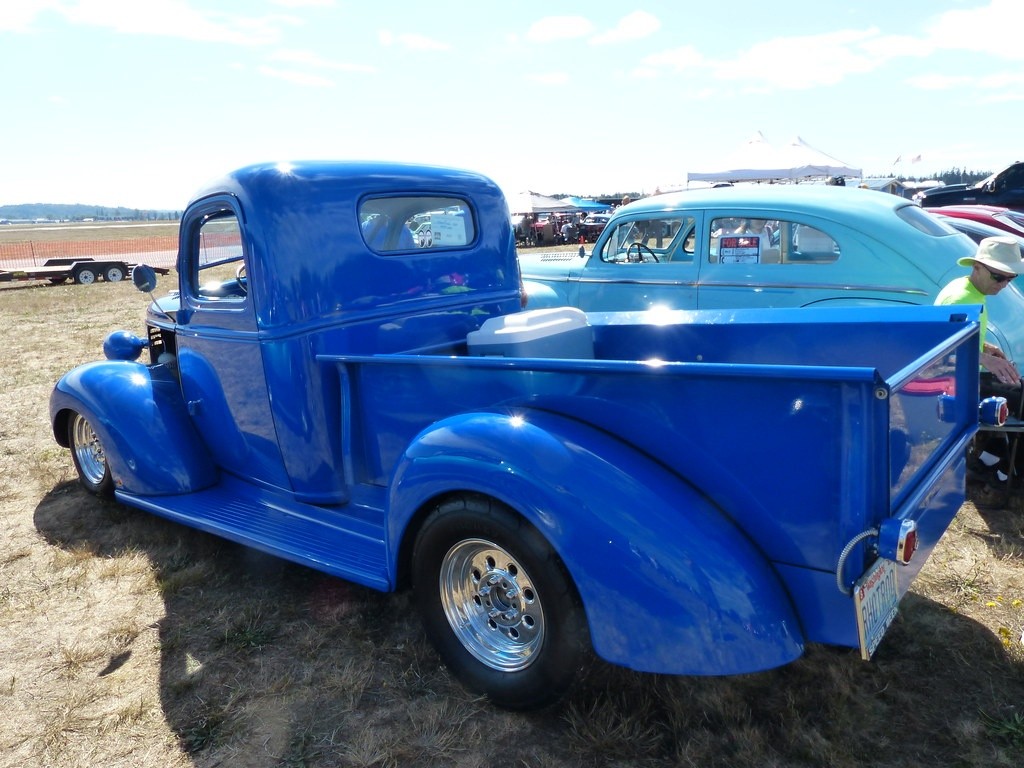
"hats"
956,234,1024,274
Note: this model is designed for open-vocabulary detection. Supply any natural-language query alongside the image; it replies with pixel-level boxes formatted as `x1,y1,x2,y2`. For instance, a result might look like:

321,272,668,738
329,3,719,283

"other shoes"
975,489,1005,507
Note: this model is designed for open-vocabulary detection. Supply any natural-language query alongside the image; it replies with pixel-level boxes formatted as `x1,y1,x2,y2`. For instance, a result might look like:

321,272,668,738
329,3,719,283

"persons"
618,195,636,247
734,218,775,250
606,203,615,214
364,212,417,250
935,233,1024,422
640,188,663,248
517,212,594,244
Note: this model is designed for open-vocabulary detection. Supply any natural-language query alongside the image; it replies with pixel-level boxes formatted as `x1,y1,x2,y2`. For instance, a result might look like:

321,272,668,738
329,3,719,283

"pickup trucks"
47,156,987,717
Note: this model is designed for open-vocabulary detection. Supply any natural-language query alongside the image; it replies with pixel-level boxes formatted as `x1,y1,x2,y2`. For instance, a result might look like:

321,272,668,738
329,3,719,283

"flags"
912,155,922,164
894,155,901,165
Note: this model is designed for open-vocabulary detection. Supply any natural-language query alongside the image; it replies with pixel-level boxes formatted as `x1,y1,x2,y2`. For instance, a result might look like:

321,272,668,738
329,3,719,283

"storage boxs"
467,307,595,360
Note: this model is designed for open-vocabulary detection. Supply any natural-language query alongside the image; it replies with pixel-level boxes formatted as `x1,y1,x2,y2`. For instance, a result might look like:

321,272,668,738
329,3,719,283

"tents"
560,195,613,223
675,128,863,190
508,190,583,245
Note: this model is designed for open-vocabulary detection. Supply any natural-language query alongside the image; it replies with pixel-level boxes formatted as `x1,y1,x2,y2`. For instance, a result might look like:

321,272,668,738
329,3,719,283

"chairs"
979,372,1024,507
759,248,779,265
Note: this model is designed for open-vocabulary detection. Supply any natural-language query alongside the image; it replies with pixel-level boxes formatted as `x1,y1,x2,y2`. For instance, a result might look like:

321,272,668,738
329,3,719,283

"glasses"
975,260,1019,281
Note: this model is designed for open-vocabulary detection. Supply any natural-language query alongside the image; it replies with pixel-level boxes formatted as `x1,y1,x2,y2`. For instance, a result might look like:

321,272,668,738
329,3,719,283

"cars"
911,157,1024,285
580,186,1022,492
504,176,913,246
